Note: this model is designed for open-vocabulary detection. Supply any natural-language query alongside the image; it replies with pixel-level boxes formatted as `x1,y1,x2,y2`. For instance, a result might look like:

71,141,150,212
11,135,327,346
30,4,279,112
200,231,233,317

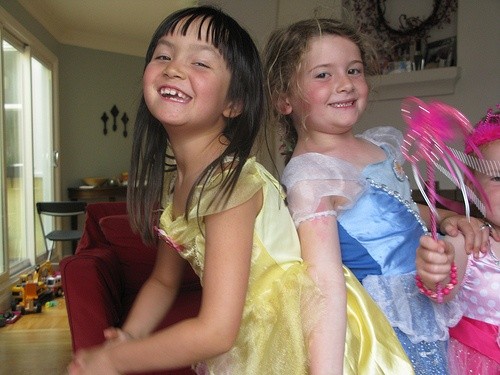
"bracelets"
415,260,457,299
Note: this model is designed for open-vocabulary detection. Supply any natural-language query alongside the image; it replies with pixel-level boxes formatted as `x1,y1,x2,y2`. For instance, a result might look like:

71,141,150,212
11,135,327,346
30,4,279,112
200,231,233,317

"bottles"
122,172,129,185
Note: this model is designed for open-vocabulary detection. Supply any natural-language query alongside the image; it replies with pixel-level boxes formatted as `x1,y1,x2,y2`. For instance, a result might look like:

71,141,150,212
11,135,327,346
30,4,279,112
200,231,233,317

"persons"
258,17,499,375
65,6,416,375
415,104,500,375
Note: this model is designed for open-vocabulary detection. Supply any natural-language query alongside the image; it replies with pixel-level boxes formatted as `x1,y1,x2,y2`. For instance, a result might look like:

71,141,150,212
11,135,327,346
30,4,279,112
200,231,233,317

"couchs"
58,201,210,375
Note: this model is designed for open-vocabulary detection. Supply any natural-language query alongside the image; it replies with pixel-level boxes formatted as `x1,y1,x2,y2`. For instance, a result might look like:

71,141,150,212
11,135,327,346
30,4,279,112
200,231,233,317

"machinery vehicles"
11,260,62,314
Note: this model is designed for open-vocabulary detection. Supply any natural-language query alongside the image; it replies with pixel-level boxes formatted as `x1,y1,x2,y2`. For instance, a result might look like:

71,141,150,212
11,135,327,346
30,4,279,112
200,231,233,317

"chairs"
35,200,87,272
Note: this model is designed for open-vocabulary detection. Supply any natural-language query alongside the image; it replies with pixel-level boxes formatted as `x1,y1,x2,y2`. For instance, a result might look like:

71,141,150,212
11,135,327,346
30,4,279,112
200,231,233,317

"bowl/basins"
81,176,108,188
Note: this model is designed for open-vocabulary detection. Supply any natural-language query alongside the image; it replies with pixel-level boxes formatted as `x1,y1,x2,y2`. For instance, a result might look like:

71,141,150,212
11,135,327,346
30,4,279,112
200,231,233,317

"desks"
67,186,147,255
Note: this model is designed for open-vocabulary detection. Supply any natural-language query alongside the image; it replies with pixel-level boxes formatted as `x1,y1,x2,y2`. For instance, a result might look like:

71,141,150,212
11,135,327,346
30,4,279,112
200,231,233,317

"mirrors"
342,0,459,102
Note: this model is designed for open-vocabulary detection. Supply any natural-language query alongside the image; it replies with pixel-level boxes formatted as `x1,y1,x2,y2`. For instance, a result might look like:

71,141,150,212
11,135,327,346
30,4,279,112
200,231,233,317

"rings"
480,225,487,231
486,223,492,228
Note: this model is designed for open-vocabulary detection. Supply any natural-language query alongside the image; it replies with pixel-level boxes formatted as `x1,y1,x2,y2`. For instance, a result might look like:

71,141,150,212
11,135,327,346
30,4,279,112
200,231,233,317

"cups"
108,179,118,187
